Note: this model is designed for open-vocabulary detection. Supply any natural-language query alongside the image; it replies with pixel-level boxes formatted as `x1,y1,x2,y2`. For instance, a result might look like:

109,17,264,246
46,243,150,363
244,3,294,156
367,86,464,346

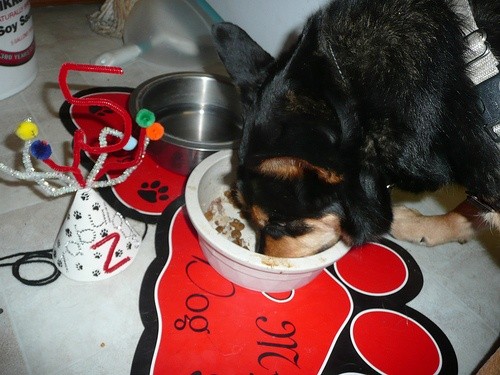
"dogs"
210,0,500,259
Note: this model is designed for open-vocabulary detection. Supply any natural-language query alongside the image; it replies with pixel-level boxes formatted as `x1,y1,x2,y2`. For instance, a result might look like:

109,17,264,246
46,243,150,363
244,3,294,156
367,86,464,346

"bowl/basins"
124,71,242,175
185,149,353,293
